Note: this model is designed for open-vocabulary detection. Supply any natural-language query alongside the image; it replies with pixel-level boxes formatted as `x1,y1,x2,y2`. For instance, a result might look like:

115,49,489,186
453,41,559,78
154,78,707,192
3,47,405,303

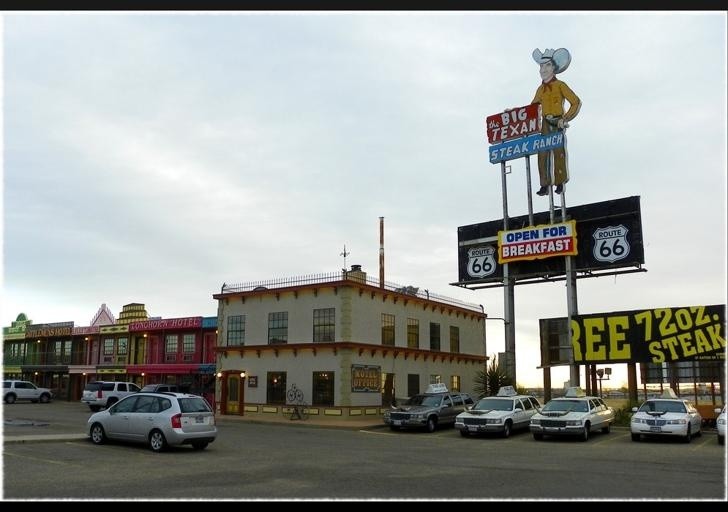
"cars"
714,404,727,444
383,383,615,441
630,388,702,443
2,380,56,404
80,381,217,452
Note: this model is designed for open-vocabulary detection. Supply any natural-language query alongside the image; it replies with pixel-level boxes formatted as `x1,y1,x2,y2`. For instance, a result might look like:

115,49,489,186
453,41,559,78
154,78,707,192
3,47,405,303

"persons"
503,47,581,195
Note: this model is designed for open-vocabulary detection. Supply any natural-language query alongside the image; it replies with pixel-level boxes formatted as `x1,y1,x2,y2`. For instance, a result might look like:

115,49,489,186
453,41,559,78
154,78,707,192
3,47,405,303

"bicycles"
287,383,304,402
